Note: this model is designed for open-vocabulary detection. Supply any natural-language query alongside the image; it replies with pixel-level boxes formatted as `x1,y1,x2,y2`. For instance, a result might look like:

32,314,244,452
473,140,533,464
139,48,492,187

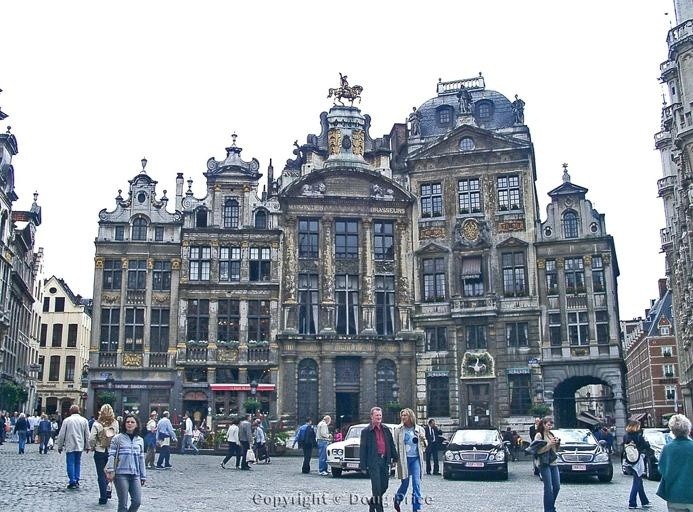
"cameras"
554,437,561,443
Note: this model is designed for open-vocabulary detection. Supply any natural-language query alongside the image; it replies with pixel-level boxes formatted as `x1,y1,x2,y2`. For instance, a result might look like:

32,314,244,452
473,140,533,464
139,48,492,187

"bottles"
104,480,112,499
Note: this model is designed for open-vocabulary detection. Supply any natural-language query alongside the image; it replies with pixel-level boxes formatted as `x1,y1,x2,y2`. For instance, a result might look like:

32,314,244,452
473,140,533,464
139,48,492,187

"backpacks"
623,440,640,465
97,427,115,447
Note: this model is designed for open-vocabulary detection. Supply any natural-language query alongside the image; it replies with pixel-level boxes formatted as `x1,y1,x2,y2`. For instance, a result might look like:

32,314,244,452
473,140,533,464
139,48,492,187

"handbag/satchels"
47,437,53,448
246,444,256,463
103,440,120,481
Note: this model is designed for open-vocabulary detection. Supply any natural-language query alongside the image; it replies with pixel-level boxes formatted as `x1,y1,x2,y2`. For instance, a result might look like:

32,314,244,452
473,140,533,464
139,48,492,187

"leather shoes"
367,497,376,512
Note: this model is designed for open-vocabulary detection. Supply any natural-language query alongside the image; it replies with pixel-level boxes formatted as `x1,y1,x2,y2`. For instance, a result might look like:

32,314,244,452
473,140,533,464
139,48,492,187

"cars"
621,426,691,481
528,426,613,485
324,421,401,479
439,424,513,479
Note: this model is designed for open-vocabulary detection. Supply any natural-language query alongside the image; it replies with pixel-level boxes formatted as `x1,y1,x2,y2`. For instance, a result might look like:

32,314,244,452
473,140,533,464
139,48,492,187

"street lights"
247,379,257,436
105,375,117,409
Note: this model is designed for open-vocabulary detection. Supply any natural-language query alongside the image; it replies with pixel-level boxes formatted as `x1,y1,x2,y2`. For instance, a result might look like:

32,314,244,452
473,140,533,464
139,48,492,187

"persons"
512,431,521,461
393,407,428,512
594,426,617,455
408,106,422,135
360,406,399,512
534,416,561,512
657,413,693,512
339,73,354,95
424,418,442,476
621,420,658,509
456,84,470,113
503,427,515,462
529,417,541,470
511,94,525,123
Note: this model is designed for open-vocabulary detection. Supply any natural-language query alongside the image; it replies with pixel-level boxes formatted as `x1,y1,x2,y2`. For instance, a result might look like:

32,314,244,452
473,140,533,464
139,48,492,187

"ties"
431,428,434,441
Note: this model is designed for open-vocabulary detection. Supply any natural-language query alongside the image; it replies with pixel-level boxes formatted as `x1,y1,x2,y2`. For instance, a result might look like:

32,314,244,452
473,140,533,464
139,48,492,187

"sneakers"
156,464,172,469
629,501,652,510
318,471,328,476
67,483,79,489
